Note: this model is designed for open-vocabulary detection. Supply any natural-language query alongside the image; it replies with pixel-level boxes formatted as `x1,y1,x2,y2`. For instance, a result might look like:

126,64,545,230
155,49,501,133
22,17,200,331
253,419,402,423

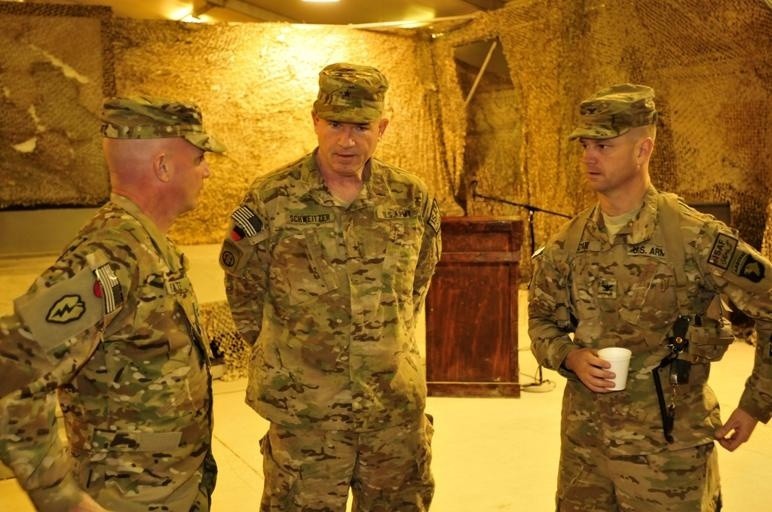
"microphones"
469,178,478,201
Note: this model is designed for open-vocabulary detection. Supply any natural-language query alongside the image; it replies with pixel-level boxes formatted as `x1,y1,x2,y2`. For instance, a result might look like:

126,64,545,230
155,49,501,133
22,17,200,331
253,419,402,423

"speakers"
683,203,733,225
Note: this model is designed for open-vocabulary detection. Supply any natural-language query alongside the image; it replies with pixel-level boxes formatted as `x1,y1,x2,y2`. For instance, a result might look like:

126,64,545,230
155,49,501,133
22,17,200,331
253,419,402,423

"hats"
565,81,661,145
311,62,390,124
98,94,231,154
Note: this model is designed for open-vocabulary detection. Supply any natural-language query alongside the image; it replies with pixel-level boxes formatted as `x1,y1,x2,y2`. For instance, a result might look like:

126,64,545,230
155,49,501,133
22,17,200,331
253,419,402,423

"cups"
596,347,633,393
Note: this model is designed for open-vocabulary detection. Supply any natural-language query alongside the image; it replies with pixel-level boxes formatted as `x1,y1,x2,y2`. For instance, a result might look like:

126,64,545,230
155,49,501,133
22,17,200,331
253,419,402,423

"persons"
1,95,231,507
217,62,443,511
526,82,772,511
